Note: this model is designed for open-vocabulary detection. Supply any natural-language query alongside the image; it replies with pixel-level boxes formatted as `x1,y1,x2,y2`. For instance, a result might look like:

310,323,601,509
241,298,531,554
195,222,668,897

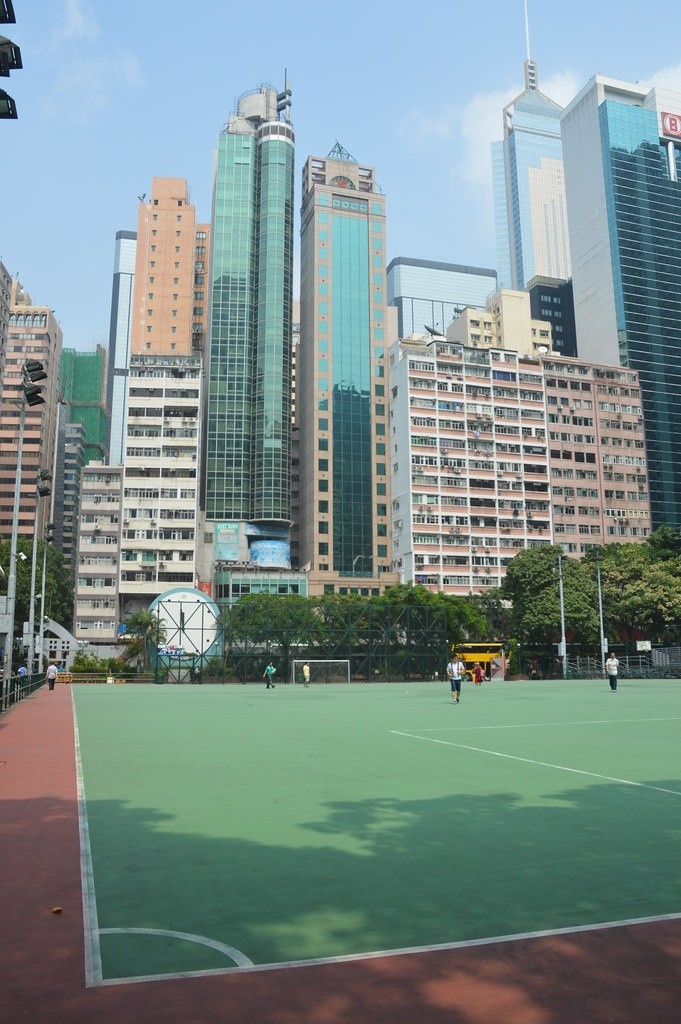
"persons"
472,662,482,686
446,654,465,703
18,665,27,689
263,661,276,689
46,661,58,690
0,647,4,661
606,653,619,691
302,662,310,688
193,666,202,684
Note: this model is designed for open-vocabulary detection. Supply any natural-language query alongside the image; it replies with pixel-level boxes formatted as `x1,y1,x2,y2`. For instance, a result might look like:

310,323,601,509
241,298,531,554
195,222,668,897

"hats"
474,661,479,665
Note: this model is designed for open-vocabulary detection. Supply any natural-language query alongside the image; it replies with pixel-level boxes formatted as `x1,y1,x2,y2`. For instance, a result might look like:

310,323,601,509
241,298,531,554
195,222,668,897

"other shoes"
612,689,616,692
457,697,460,703
453,697,456,702
272,686,275,688
266,687,269,688
